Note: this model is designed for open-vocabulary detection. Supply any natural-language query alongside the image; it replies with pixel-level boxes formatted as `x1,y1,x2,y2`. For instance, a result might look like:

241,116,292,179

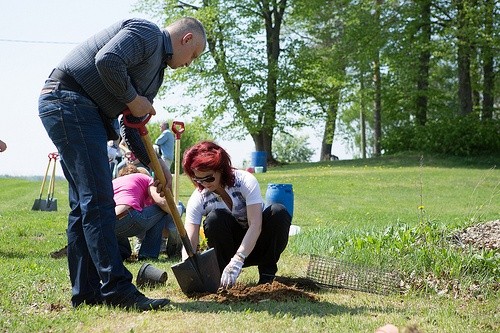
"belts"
116,206,134,220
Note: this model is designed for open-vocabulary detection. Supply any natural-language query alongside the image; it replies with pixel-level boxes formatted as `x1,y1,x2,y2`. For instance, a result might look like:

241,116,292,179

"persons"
181,140,292,289
0,140,7,152
134,166,168,257
155,121,175,170
111,164,173,261
107,133,159,178
38,16,206,311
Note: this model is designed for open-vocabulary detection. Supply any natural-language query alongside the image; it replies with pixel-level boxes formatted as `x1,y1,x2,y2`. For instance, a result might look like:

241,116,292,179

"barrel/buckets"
251,151,267,173
136,264,167,287
255,167,263,173
266,183,294,222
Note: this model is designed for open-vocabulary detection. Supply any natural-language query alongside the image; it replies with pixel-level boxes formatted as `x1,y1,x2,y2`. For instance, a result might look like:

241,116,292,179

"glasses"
192,174,215,183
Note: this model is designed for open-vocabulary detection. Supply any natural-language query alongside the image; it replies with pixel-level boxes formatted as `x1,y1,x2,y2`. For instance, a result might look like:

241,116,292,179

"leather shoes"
123,290,170,312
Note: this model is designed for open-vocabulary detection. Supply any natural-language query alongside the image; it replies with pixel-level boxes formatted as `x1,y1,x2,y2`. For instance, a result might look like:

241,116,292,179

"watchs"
236,252,246,260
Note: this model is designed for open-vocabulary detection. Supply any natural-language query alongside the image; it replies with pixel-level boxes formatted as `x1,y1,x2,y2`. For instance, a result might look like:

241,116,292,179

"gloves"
221,254,244,289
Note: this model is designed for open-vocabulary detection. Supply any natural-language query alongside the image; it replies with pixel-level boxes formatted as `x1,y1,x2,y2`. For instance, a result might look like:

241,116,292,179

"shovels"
124,110,221,299
32,152,59,211
172,122,182,217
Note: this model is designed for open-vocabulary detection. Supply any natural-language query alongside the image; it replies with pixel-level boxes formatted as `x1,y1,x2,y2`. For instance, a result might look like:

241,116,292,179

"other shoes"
258,274,275,284
